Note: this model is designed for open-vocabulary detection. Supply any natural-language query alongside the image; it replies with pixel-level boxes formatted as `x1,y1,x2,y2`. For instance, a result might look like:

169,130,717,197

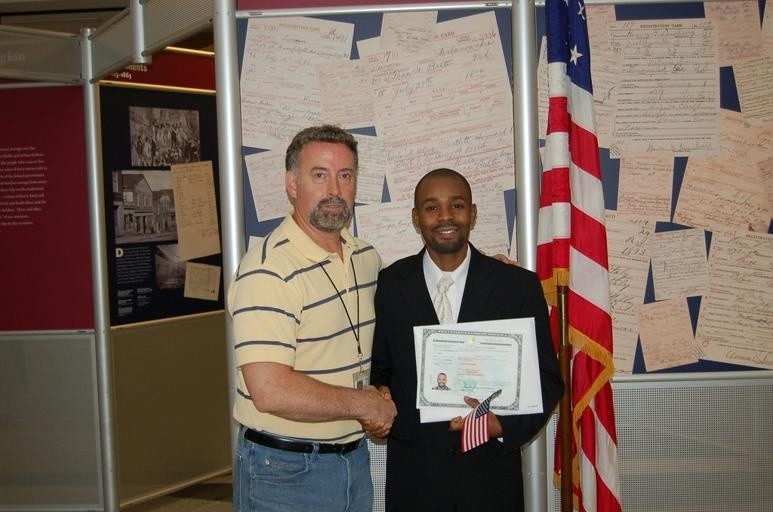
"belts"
238,428,360,455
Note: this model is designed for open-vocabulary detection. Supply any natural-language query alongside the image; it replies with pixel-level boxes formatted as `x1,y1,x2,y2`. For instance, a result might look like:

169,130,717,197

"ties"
433,274,454,325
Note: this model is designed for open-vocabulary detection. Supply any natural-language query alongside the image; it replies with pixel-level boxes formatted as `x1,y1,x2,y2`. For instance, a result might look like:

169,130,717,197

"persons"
134,121,200,167
432,371,450,391
358,382,363,390
225,120,397,511
359,165,568,512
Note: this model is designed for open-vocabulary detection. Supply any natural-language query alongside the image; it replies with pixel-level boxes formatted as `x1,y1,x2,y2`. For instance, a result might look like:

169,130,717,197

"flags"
459,389,501,453
534,0,623,510
